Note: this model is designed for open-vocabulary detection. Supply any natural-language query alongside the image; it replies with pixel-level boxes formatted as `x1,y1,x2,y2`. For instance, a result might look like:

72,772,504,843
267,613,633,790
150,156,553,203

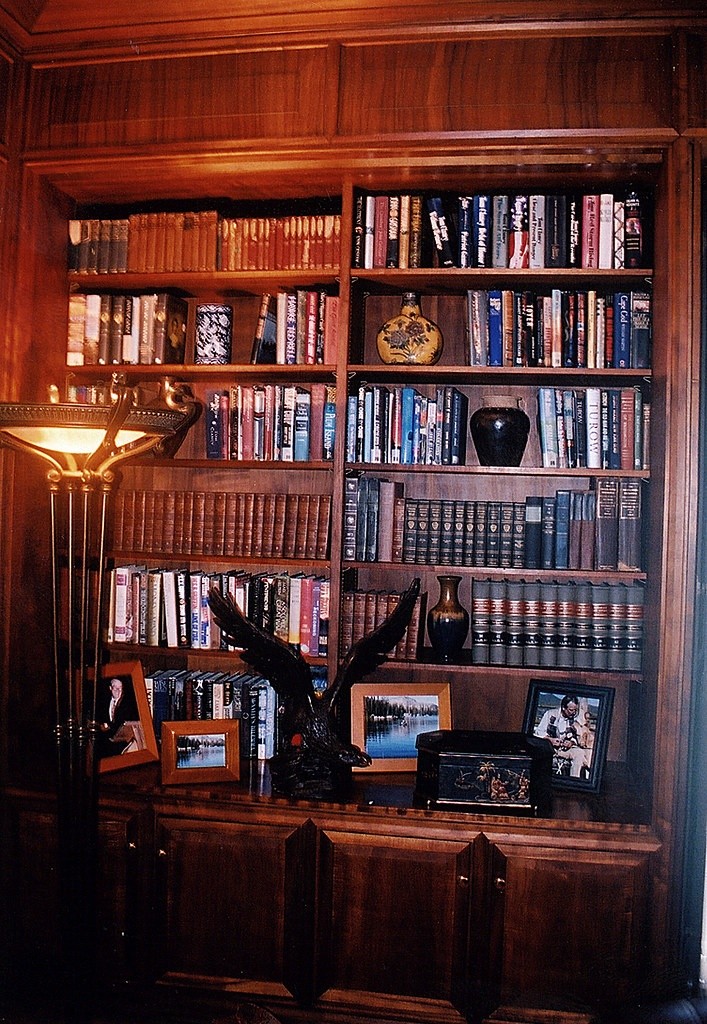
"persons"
537,696,595,779
95,678,138,760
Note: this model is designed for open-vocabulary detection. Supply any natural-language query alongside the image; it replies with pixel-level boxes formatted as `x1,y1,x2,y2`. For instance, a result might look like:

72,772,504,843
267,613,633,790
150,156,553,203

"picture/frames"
415,725,557,816
522,675,619,795
66,661,159,779
349,681,455,772
163,718,241,786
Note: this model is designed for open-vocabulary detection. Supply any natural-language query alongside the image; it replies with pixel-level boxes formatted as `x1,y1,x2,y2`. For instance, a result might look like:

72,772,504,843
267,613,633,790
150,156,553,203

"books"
342,476,646,572
343,575,646,672
141,667,330,759
67,287,338,364
346,381,651,465
466,290,652,370
65,207,346,275
114,487,331,559
66,384,336,463
109,564,329,658
352,192,651,268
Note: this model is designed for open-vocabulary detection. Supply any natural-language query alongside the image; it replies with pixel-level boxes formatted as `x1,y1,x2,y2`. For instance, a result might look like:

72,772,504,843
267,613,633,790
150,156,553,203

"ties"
112,700,116,721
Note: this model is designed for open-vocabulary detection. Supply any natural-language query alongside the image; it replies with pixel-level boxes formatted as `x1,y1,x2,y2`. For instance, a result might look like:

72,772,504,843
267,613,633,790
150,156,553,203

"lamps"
0,367,205,1023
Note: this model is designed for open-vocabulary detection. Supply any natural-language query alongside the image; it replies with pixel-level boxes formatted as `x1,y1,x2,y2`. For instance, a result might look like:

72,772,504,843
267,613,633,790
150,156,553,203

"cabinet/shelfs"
5,127,702,1023
